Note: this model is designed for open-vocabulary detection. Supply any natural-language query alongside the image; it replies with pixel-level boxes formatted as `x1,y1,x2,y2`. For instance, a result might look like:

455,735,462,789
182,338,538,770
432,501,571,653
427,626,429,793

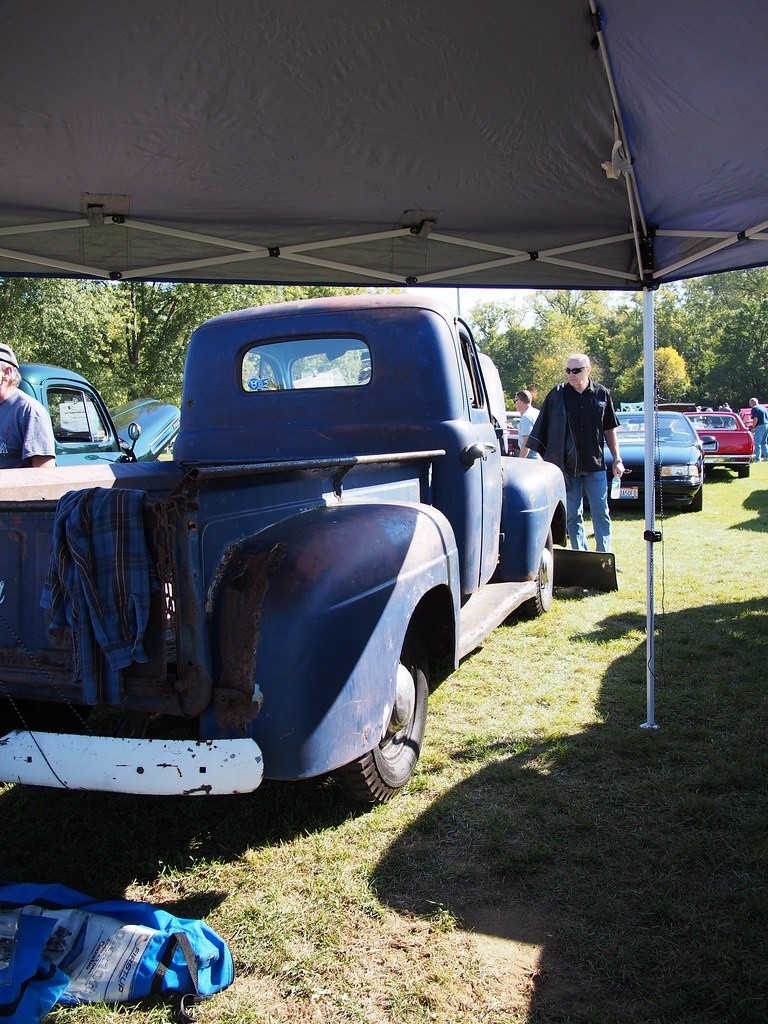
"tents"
0,0,768,729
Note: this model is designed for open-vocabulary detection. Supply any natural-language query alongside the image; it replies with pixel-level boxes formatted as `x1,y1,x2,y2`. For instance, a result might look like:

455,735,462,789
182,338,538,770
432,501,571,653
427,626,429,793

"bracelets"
613,458,622,462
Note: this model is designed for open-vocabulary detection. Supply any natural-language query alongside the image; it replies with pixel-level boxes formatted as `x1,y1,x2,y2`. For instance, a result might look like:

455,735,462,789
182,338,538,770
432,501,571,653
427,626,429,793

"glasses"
566,366,588,374
514,398,522,404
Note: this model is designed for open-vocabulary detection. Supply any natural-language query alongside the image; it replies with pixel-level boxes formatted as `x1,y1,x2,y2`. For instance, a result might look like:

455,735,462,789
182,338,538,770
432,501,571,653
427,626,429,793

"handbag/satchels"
0,882,235,1024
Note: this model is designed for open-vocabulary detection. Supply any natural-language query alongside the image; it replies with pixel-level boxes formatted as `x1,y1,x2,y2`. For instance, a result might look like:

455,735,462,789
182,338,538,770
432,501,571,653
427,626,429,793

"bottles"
611,473,621,500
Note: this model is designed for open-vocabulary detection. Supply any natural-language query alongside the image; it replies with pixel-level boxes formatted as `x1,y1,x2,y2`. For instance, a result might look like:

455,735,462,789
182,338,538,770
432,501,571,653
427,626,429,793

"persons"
0,344,56,469
706,408,724,425
525,355,625,572
749,398,768,463
516,391,543,459
719,403,736,428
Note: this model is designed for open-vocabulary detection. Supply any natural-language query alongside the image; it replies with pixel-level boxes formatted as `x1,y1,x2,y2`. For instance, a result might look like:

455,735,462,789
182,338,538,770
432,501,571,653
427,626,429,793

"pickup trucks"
1,295,567,805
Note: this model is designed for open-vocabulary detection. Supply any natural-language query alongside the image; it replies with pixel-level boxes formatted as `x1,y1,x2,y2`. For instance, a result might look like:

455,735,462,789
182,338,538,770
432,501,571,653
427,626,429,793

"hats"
0,343,20,368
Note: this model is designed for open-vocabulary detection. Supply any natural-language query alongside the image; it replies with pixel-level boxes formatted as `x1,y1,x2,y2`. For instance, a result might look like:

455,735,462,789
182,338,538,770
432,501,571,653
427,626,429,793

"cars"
583,411,702,513
14,362,180,467
684,411,755,478
